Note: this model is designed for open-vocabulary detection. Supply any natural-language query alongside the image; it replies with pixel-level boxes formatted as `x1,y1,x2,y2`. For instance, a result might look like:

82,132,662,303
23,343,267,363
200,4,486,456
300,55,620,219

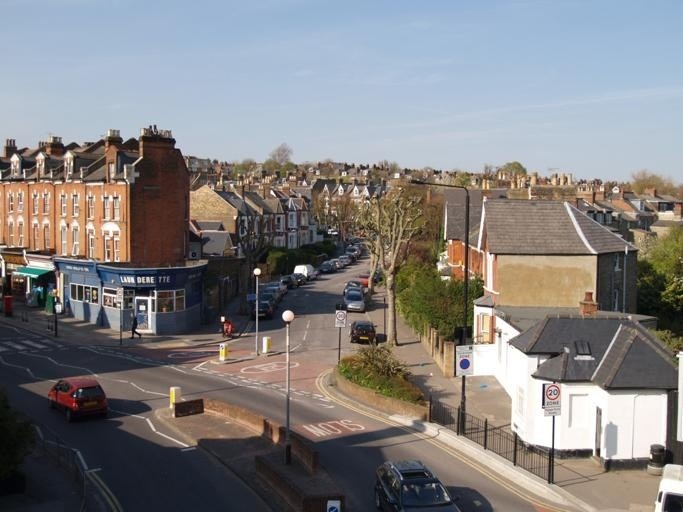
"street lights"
253,268,261,356
281,309,295,464
408,179,470,436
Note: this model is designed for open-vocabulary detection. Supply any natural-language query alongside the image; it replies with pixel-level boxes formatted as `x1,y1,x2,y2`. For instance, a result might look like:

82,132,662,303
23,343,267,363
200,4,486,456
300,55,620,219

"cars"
47,377,108,423
249,273,307,319
319,225,363,275
348,320,377,344
342,273,369,313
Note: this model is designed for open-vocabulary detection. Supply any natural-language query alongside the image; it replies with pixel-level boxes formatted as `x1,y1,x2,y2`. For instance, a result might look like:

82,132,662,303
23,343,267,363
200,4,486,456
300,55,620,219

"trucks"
655,463,682,512
294,264,318,281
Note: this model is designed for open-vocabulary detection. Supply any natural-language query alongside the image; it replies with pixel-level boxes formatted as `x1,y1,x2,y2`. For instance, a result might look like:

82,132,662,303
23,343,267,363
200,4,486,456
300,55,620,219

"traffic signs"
545,384,561,416
335,310,346,328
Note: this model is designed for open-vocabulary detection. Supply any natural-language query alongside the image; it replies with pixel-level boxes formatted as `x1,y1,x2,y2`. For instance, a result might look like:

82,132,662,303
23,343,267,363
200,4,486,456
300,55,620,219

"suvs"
374,458,461,512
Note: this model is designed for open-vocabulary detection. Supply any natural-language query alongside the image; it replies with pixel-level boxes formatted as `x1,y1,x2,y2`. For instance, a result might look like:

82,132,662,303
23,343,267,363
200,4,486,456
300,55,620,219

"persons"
129,313,143,339
